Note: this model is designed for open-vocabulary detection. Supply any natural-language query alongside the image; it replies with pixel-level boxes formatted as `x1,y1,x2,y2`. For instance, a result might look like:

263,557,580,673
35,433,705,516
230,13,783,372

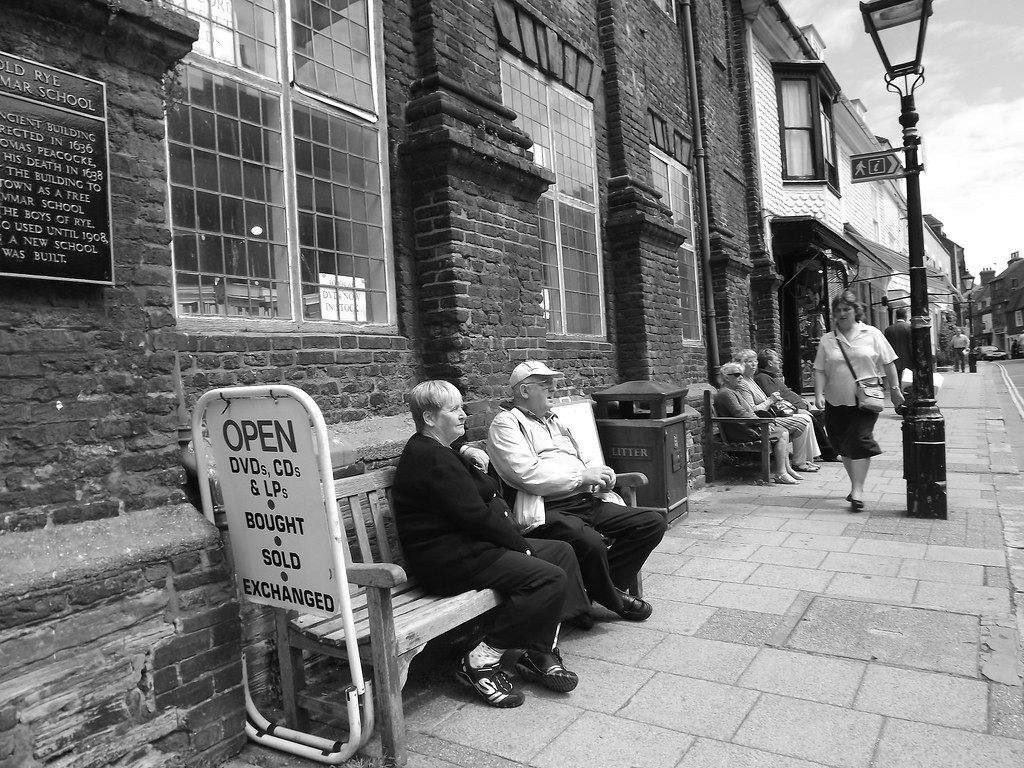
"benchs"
704,390,776,484
278,469,649,768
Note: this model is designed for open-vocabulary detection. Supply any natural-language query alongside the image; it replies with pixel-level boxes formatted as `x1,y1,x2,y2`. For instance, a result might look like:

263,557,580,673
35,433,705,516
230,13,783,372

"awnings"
771,216,860,289
846,229,966,308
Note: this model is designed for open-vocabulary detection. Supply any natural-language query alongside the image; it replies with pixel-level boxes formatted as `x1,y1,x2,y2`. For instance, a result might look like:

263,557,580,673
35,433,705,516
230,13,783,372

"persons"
486,362,666,631
393,380,579,708
1011,330,1024,358
736,349,821,471
945,328,969,372
814,292,906,511
714,363,804,485
755,348,841,461
884,309,914,402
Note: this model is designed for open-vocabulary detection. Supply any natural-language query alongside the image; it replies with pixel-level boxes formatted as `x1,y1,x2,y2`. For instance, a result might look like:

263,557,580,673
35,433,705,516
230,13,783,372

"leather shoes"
565,612,593,631
615,588,653,621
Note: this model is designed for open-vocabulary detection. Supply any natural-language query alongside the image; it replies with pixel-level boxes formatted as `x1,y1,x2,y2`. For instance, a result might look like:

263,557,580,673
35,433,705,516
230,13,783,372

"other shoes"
823,454,843,462
846,489,863,502
791,460,820,472
851,499,863,508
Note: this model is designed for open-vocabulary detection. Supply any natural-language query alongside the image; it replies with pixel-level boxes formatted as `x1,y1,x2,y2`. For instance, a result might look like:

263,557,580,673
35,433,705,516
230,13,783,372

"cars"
973,346,1008,361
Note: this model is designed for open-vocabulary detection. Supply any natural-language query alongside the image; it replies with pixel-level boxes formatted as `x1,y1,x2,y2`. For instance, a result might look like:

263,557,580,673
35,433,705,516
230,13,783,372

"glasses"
726,372,743,377
521,380,554,388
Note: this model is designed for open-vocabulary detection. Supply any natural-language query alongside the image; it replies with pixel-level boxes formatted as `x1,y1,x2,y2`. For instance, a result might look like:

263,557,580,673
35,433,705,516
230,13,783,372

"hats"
509,360,564,388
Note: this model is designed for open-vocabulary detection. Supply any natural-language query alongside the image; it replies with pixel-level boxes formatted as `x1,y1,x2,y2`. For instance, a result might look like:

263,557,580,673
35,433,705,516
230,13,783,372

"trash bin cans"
591,379,691,530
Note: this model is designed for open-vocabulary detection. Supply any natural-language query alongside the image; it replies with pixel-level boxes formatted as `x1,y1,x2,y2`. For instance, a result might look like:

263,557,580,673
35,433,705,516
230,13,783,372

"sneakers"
516,648,579,693
455,652,525,708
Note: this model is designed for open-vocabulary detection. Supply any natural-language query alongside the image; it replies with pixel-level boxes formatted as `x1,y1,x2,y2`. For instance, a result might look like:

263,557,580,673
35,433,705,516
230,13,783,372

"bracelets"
890,386,898,390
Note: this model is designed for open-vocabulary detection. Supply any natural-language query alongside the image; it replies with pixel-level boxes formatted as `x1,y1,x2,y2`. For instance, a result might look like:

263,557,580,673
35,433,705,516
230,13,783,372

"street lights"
859,0,948,518
961,270,977,373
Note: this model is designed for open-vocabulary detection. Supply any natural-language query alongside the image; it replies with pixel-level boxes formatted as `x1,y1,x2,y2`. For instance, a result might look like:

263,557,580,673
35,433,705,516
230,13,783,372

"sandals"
774,473,800,485
787,469,804,480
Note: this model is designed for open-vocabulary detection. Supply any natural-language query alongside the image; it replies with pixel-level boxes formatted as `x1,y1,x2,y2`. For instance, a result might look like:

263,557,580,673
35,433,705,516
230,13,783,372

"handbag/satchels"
855,385,884,411
770,396,797,417
